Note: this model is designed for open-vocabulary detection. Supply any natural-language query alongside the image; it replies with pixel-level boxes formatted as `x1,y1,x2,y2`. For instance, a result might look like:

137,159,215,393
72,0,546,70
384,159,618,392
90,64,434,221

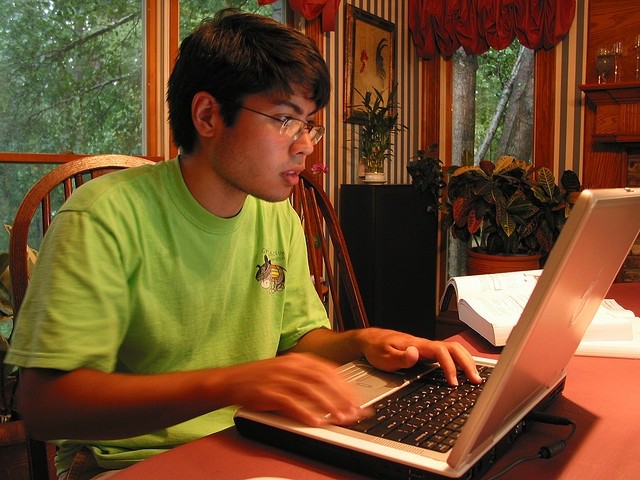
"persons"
3,7,483,480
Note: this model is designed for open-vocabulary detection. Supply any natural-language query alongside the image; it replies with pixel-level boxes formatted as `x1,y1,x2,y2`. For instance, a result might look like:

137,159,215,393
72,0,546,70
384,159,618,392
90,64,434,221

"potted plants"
344,83,412,186
442,156,583,274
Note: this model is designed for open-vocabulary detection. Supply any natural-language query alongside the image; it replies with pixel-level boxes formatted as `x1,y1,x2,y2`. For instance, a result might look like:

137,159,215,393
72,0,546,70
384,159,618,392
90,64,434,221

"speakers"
331,183,439,341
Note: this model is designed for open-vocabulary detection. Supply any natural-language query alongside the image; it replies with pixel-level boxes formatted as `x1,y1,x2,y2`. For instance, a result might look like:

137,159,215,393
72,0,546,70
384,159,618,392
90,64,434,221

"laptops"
233,187,640,479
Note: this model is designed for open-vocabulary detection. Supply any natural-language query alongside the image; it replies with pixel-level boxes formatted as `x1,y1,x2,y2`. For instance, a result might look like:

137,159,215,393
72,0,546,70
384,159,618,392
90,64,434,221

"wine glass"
610,40,625,83
595,47,612,85
633,35,640,82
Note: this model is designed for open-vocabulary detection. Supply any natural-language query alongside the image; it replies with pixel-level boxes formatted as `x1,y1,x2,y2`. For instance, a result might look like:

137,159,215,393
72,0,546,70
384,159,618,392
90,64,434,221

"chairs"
288,171,369,332
10,155,158,480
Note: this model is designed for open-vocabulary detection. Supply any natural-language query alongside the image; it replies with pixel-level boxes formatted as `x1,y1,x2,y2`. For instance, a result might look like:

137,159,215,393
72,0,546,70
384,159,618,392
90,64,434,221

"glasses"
215,97,325,146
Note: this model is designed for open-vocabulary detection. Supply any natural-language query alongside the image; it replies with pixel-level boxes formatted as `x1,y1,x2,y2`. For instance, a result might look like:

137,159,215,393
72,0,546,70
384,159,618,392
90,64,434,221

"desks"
106,280,640,480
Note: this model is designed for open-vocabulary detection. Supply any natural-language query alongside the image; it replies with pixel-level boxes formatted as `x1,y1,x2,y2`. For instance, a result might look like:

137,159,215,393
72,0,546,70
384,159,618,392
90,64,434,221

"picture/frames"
343,5,398,125
